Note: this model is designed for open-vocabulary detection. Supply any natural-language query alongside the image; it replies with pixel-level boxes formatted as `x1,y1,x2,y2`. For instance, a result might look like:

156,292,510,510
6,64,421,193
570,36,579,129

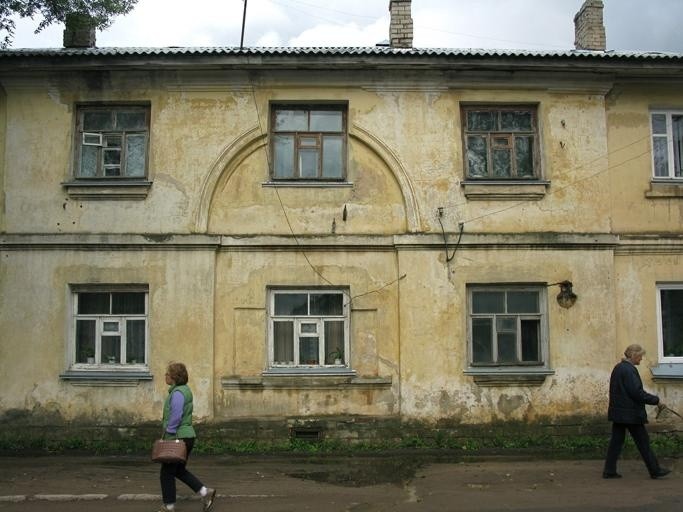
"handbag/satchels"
151,429,188,464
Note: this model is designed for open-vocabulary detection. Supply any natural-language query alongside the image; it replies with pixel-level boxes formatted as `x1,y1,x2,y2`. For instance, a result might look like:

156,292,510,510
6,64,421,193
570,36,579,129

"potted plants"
105,348,119,364
84,346,94,364
130,352,139,364
329,348,344,366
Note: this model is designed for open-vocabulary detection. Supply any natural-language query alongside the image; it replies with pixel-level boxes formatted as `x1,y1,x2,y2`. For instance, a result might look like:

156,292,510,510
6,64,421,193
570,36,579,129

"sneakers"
156,505,176,512
200,487,217,512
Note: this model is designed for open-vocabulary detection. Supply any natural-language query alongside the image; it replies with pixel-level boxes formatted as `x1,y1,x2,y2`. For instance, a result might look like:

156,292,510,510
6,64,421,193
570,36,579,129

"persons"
159,362,216,510
601,343,672,479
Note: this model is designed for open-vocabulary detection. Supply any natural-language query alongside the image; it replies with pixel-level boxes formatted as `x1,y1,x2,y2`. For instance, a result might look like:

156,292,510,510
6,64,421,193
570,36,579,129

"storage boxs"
64,26,95,48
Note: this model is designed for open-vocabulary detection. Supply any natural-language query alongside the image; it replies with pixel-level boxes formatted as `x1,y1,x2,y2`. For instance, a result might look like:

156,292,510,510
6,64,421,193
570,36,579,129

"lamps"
543,281,577,310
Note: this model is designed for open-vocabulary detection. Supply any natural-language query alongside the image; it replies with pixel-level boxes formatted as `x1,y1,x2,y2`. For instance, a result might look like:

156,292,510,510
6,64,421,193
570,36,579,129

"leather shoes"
651,468,669,479
603,472,623,478
